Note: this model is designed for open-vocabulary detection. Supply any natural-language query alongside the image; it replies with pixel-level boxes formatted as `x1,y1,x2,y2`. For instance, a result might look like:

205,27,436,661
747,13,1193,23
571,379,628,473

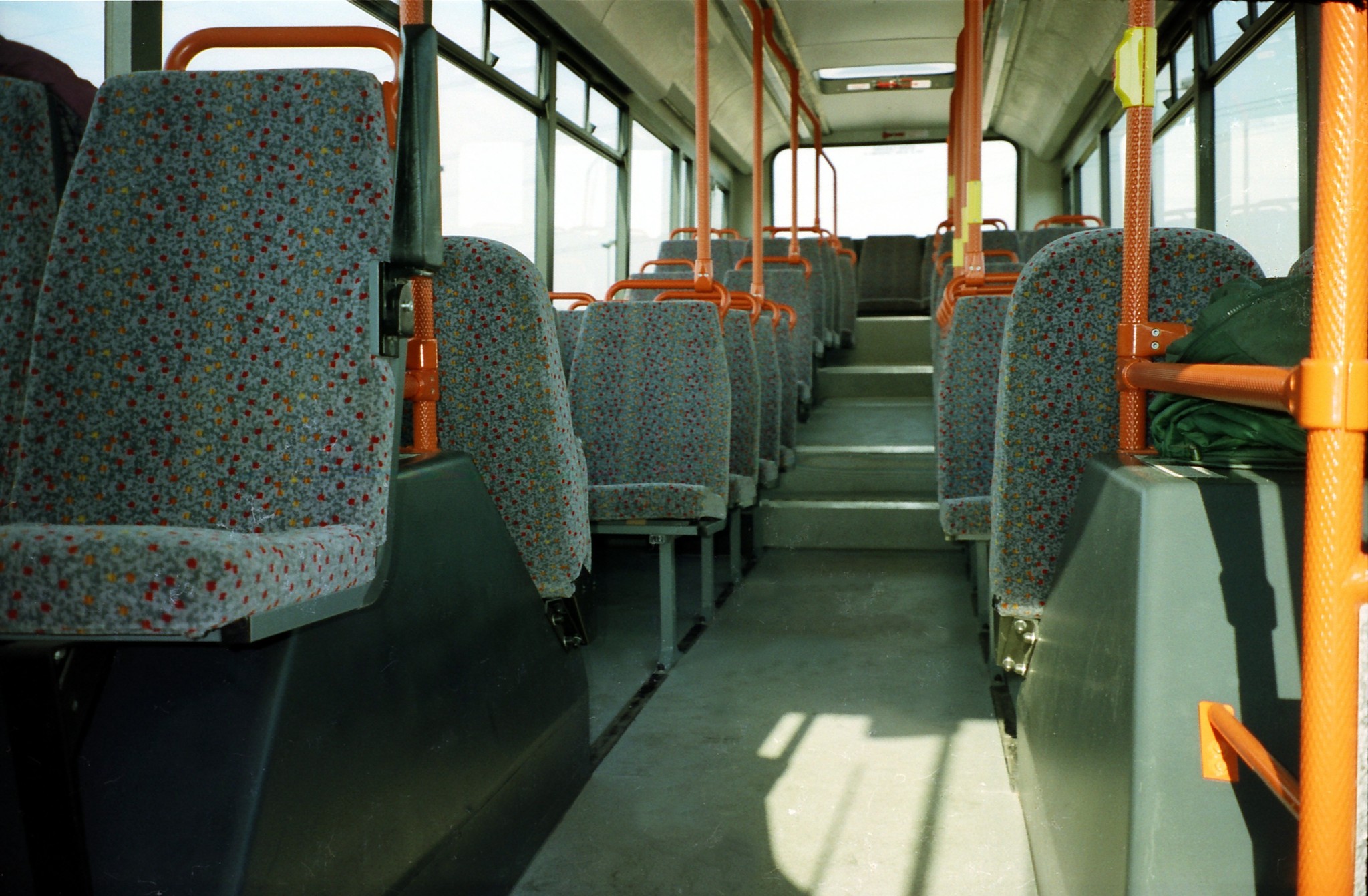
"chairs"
0,22,405,656
428,216,1314,683
